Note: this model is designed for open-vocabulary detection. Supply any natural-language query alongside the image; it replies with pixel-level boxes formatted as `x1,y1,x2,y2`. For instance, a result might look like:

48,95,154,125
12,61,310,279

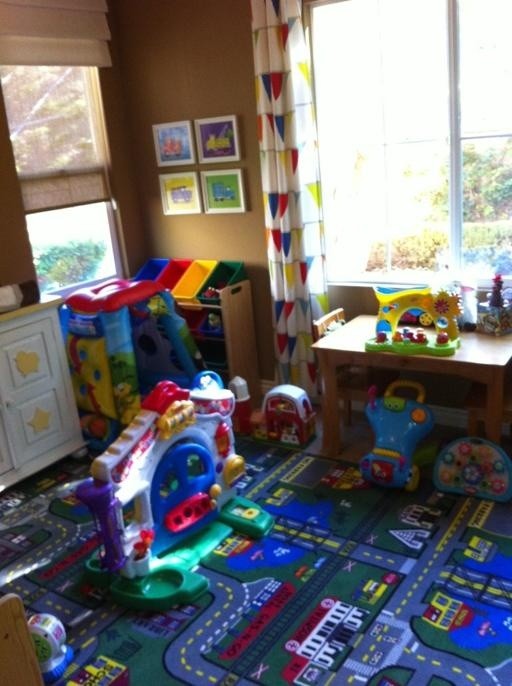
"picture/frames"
157,171,203,216
199,167,247,214
194,115,241,164
152,120,197,168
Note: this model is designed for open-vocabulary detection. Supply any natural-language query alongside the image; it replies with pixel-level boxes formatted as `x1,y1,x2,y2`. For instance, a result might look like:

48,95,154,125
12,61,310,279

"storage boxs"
132,258,244,368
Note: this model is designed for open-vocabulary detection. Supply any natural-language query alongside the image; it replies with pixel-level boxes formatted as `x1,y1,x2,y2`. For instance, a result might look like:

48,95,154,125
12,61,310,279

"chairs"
467,382,512,437
312,308,400,448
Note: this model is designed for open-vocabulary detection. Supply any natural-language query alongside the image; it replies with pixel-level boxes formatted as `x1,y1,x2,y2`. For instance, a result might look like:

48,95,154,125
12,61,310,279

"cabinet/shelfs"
163,280,260,409
0,294,90,496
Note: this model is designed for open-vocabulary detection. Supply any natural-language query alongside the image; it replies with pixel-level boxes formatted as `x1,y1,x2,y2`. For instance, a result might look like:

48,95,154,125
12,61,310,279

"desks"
311,313,512,456
